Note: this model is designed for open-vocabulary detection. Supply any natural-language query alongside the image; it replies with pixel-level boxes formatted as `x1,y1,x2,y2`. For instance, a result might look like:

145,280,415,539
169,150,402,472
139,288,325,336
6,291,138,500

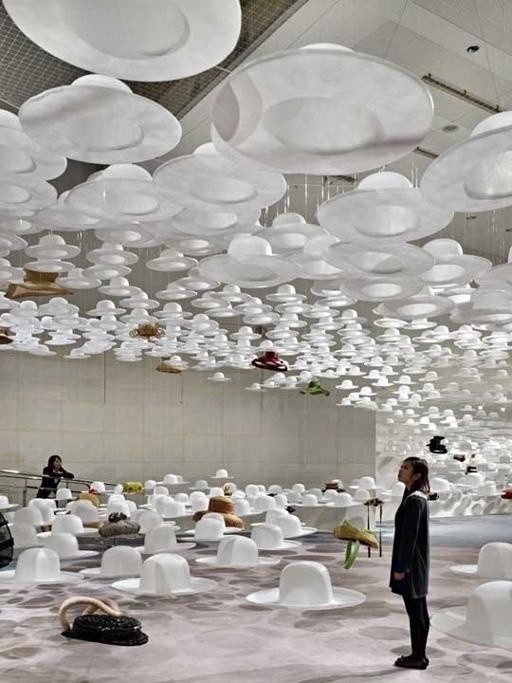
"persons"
387,456,430,669
37,455,75,498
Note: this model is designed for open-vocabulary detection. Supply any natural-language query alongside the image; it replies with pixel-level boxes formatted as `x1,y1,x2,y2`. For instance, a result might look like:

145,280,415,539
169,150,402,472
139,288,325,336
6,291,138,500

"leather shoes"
394,654,430,670
